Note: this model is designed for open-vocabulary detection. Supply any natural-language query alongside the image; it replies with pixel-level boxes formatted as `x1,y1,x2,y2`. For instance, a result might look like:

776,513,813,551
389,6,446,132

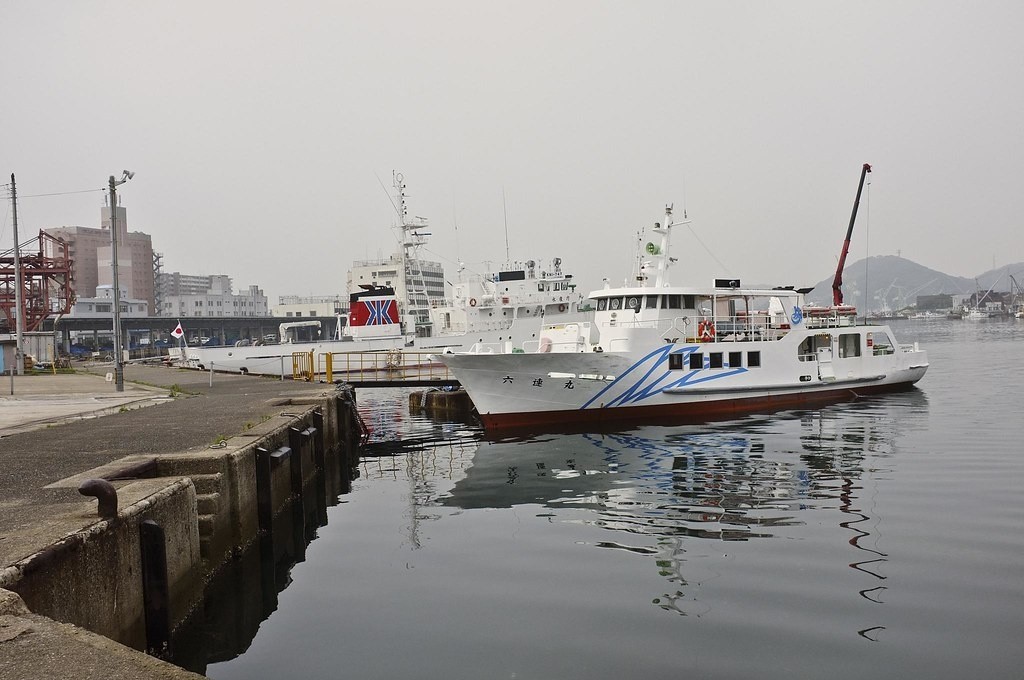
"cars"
193,336,210,344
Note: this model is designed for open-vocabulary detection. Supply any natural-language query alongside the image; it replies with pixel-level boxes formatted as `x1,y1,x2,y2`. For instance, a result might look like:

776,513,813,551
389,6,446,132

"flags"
171,323,185,339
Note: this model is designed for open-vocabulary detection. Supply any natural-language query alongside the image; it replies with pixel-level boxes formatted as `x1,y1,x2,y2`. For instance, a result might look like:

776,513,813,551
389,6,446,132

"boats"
425,163,931,437
962,310,989,320
165,166,596,385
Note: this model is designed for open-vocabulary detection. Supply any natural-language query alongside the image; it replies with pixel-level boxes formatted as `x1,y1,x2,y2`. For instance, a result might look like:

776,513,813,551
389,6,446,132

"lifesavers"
558,304,565,312
469,298,477,306
698,320,715,342
